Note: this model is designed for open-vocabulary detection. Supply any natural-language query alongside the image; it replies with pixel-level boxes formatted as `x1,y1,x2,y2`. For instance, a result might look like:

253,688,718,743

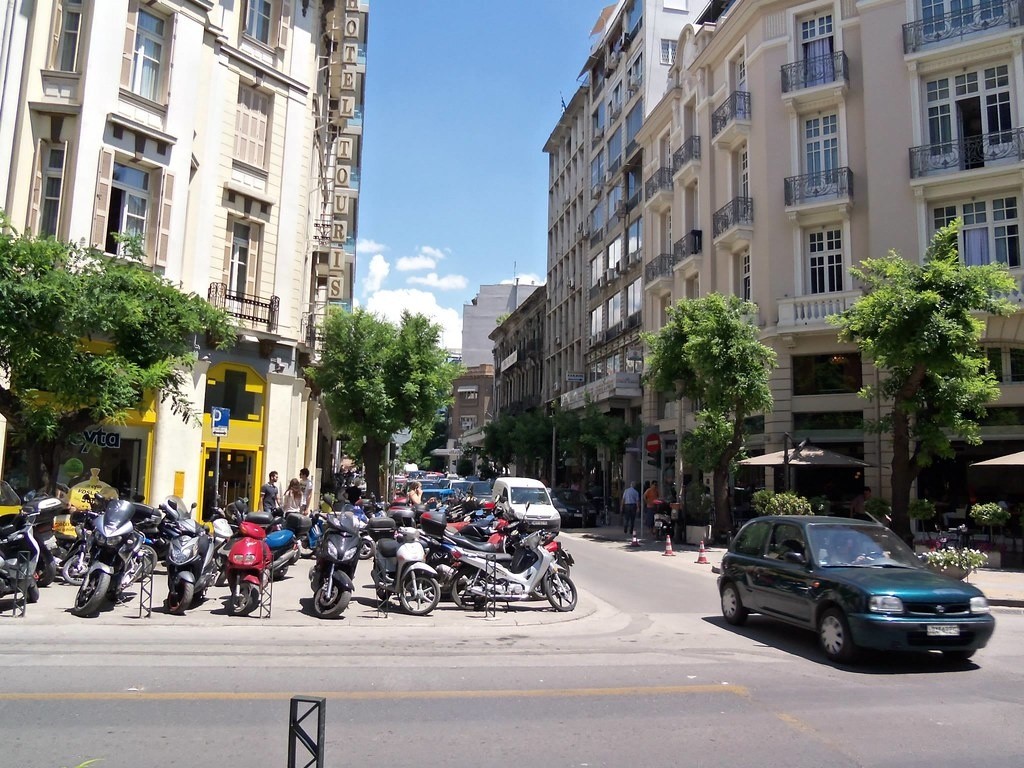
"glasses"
653,484,658,486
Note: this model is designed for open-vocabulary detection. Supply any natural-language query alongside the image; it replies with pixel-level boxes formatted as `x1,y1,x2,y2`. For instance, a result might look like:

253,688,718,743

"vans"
491,477,560,535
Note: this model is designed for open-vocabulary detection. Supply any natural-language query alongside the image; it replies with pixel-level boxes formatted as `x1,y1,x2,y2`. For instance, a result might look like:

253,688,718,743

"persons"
620,480,639,536
406,481,423,505
831,533,871,565
570,481,580,491
643,478,677,538
258,471,284,513
339,464,362,506
965,489,1008,533
850,485,872,521
283,468,313,520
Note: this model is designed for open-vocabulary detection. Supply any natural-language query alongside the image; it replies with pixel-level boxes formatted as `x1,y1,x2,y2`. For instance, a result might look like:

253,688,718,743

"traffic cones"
662,535,675,556
630,528,640,547
694,541,709,564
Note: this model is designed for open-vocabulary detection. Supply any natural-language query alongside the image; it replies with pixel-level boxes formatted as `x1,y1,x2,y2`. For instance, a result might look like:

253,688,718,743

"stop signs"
645,434,660,451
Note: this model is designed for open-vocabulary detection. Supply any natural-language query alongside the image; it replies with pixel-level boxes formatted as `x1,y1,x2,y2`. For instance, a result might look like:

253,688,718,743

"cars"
465,482,493,509
393,471,453,504
426,473,479,492
718,515,996,664
546,488,597,527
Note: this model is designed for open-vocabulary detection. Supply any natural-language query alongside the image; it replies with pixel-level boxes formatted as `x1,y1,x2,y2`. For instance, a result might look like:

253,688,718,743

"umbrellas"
970,450,1024,467
736,443,891,467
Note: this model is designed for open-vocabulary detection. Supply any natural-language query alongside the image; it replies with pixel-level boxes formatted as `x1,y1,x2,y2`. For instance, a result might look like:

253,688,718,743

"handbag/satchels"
272,508,284,518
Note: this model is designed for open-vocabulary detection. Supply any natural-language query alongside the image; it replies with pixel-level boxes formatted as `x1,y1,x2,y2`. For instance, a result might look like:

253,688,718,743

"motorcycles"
310,485,387,618
418,493,574,600
63,491,165,616
0,480,62,603
652,499,672,540
367,518,440,616
442,529,577,611
128,496,232,614
223,498,311,614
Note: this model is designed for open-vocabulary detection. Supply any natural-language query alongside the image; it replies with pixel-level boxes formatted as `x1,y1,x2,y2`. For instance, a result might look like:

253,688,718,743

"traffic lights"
647,451,656,466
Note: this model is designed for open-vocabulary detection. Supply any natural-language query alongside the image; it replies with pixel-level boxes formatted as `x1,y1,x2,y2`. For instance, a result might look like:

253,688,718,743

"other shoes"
624,528,627,533
630,528,633,533
